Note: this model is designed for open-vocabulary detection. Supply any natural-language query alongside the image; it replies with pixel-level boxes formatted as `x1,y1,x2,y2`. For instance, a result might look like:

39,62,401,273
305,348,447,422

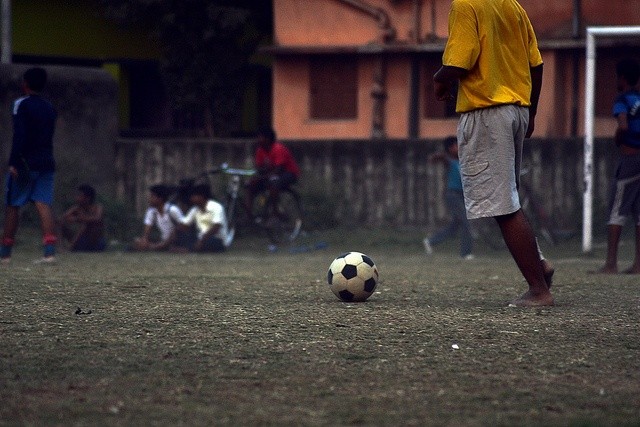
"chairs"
32,255,57,267
0,256,11,263
423,237,432,255
459,254,472,260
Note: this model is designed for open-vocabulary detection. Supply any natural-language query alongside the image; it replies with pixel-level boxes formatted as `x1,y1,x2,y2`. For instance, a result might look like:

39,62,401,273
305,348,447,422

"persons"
54,185,107,251
234,126,300,229
432,0,555,306
127,184,185,252
586,57,640,273
0,67,57,267
422,136,475,259
167,183,229,252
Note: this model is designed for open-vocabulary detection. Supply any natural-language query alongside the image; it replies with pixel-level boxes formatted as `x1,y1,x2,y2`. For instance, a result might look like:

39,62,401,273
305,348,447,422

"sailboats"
329,250,379,302
